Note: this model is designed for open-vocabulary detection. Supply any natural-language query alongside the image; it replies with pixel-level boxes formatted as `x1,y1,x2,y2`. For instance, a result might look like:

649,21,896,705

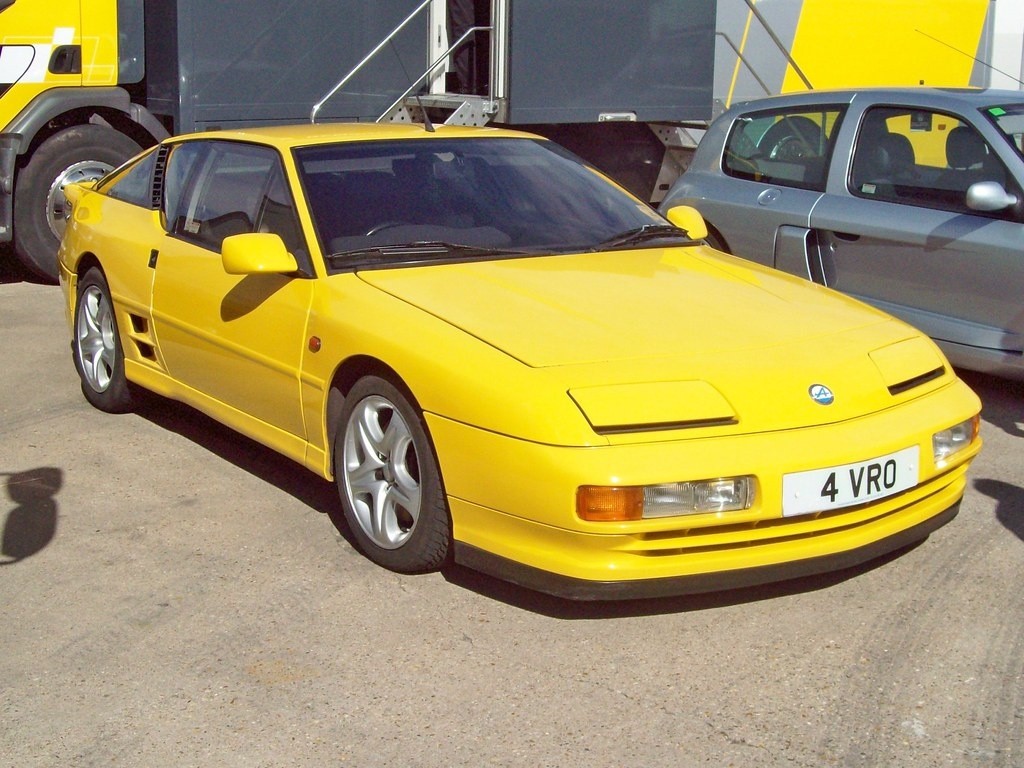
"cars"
662,89,1024,391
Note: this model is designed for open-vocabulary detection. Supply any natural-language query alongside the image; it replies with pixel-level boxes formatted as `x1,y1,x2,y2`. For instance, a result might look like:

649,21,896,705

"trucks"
0,0,1023,285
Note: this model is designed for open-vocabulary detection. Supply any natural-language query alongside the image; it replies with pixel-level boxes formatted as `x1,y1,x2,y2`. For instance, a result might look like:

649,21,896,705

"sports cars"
58,120,984,602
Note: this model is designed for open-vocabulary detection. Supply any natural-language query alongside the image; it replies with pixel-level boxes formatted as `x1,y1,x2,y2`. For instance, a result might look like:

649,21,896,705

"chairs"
869,132,948,206
938,126,1009,206
